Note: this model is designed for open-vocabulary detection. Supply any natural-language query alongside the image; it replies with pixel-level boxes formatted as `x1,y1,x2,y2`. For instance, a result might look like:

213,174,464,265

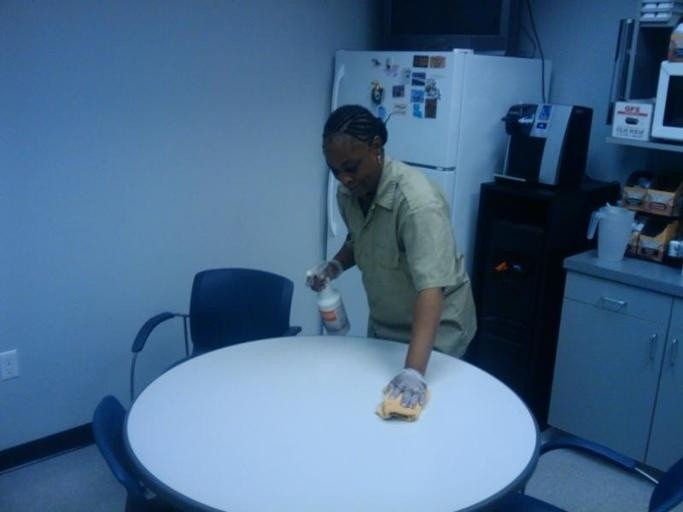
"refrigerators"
322,49,553,336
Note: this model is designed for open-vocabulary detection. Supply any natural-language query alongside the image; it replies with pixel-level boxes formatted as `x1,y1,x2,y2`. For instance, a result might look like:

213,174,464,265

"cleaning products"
316,276,352,336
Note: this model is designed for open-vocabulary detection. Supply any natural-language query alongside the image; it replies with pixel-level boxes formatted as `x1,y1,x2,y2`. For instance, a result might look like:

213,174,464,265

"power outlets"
0,350,20,381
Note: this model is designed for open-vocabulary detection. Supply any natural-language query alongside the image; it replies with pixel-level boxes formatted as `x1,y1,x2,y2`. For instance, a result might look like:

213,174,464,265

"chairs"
129,268,302,401
479,435,682,512
92,394,175,512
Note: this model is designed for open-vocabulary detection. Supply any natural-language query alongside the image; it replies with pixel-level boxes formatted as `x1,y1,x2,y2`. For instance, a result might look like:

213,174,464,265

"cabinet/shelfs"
548,250,683,471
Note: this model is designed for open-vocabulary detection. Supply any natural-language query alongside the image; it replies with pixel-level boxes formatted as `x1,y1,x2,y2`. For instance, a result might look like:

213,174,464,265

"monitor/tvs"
651,60,683,145
382,0,512,56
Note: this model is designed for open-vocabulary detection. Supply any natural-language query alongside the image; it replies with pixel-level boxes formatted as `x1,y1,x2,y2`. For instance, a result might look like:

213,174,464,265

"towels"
374,387,431,422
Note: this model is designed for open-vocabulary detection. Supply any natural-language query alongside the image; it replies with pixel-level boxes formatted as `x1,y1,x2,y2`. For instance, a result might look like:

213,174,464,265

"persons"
303,104,478,411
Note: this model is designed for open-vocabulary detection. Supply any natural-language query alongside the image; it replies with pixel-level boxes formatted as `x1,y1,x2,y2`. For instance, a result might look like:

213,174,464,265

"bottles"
667,216,683,268
